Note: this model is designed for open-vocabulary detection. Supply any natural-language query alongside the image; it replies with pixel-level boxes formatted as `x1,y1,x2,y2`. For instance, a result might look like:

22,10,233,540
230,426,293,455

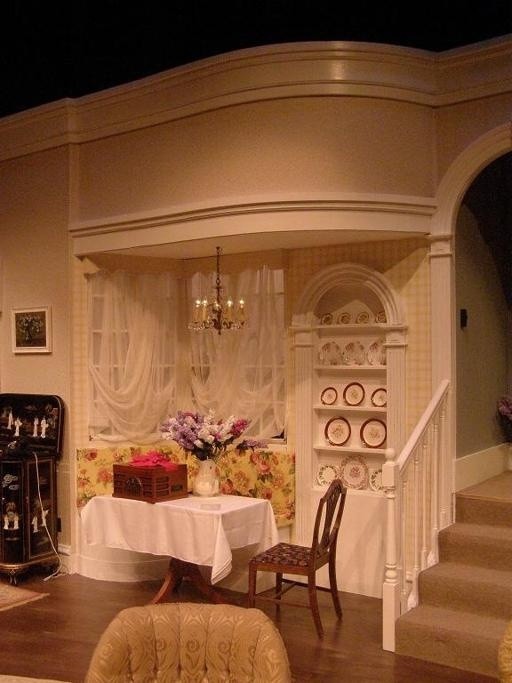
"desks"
92,493,271,605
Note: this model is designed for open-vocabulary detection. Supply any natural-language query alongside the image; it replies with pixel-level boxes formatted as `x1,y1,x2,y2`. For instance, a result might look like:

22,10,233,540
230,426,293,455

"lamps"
185,245,248,334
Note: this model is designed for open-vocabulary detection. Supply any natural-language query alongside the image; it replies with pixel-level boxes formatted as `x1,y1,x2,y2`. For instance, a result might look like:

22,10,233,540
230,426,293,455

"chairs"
84,602,292,683
247,479,347,639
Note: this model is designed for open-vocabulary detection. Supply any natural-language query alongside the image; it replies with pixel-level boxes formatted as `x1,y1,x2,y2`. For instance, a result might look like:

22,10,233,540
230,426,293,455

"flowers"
160,408,270,461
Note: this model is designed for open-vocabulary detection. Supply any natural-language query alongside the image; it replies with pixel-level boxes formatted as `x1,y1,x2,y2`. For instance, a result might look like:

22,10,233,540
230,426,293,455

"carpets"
0,582,51,612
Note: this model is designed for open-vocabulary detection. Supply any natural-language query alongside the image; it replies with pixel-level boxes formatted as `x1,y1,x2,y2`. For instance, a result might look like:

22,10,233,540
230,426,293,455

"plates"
321,313,333,325
356,312,370,324
319,341,342,366
370,388,387,406
367,339,386,365
337,311,352,324
339,454,370,490
369,469,382,491
324,415,352,446
375,310,386,323
317,465,339,486
343,382,365,406
359,418,387,449
321,386,338,404
343,341,366,367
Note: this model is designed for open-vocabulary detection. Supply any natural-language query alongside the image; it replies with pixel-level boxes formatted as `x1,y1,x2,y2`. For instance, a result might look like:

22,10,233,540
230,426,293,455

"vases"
192,458,221,496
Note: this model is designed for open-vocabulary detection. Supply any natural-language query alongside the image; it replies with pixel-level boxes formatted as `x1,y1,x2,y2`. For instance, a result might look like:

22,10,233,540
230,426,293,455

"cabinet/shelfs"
0,392,63,586
311,321,388,497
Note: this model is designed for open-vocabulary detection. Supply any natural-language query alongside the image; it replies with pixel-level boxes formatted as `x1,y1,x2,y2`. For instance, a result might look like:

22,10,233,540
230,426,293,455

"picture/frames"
9,303,53,353
